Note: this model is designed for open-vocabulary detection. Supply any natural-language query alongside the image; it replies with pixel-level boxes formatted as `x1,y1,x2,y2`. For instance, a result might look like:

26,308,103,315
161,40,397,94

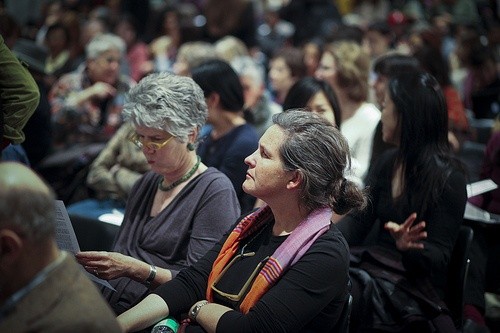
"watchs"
192,301,210,321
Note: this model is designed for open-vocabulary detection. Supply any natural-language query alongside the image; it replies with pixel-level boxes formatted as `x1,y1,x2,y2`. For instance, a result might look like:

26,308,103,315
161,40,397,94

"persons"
0,32,41,169
0,160,124,333
114,110,369,333
368,0,500,247
75,70,241,317
0,0,382,215
335,56,471,333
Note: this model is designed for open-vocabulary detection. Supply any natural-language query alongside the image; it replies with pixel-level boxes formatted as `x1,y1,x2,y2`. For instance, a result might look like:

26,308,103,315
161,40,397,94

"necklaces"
159,156,201,191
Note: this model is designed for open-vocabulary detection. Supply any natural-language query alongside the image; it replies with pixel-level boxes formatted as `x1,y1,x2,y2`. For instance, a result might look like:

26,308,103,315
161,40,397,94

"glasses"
128,130,174,154
210,251,271,302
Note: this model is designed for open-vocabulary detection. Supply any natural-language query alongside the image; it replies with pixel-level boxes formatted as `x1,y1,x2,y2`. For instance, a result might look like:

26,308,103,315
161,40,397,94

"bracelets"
145,264,157,283
